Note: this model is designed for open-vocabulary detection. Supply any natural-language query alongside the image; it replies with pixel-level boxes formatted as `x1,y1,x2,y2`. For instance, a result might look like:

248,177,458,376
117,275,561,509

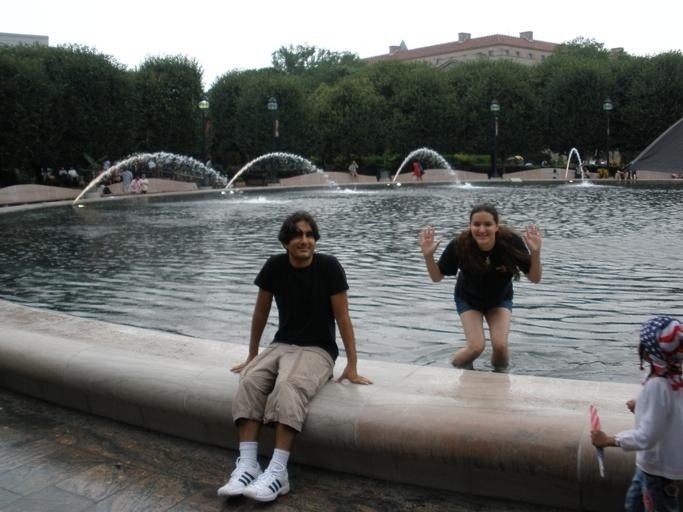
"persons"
216,210,373,502
413,159,425,181
348,160,359,183
418,205,543,368
39,159,149,194
590,315,683,512
598,165,637,180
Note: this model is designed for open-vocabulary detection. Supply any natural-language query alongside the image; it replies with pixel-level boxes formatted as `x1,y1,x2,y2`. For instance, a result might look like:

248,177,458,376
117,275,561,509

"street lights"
261,93,281,152
488,97,504,181
195,93,216,188
602,94,614,175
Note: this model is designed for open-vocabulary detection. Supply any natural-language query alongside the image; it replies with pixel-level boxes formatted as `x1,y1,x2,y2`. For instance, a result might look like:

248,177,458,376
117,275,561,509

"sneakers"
245,464,291,502
217,464,261,497
